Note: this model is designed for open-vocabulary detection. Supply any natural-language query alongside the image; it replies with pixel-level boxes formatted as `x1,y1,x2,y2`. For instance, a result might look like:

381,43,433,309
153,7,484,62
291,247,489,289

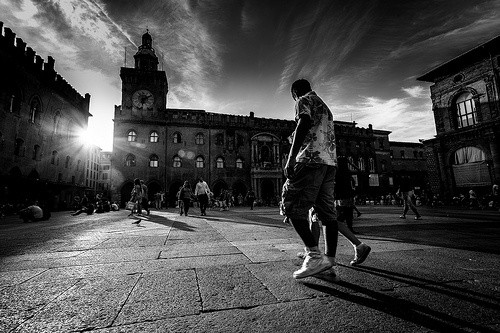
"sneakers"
293,255,333,279
313,266,339,281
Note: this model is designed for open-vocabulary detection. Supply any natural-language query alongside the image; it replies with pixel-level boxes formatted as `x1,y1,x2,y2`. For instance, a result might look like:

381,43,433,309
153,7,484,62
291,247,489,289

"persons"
215,189,243,211
399,171,421,220
70,192,119,216
151,190,169,210
297,156,371,266
195,177,213,216
354,202,362,217
245,187,256,210
128,178,142,217
178,180,196,217
177,187,184,218
136,179,150,216
282,79,338,278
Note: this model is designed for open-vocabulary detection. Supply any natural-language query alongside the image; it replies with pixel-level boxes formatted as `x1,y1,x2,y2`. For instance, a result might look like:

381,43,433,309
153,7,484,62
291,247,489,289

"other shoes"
350,244,371,266
328,257,336,265
400,216,406,219
414,216,422,220
284,219,290,223
